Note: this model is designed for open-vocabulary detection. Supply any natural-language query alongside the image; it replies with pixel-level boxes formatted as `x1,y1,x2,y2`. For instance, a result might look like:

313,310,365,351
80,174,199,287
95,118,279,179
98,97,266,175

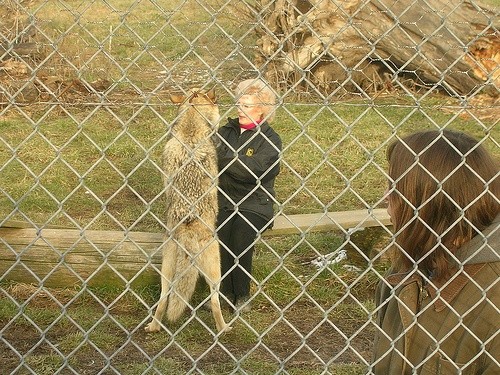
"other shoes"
202,297,234,311
234,295,251,314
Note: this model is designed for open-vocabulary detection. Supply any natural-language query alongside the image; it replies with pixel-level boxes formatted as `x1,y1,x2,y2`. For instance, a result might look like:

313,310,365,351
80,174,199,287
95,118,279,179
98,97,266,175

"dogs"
143,83,234,334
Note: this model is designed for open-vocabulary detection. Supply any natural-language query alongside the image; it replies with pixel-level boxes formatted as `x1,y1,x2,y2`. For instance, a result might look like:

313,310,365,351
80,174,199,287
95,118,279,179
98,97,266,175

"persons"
203,79,282,314
370,130,500,375
